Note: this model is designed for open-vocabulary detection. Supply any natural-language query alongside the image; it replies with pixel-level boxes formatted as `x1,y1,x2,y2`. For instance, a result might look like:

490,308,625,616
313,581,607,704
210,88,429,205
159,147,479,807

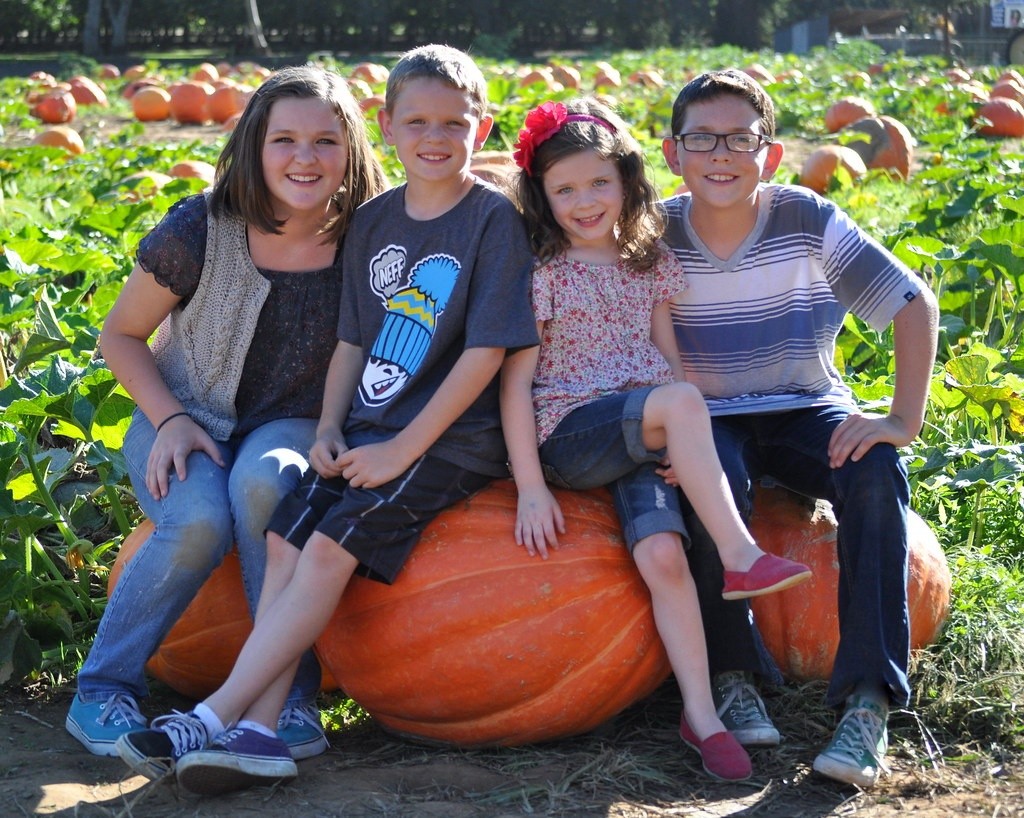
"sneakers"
812,694,891,787
710,669,780,746
276,701,331,759
115,708,210,785
65,691,147,757
175,728,298,797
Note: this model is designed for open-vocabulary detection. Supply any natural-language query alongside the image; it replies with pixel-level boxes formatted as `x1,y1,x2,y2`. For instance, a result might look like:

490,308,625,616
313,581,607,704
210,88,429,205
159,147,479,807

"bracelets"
156,410,187,434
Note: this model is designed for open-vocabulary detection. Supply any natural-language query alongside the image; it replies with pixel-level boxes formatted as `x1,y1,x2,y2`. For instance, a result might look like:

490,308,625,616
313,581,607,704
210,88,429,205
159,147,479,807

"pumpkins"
314,473,675,749
108,514,258,699
22,59,1024,204
745,481,952,684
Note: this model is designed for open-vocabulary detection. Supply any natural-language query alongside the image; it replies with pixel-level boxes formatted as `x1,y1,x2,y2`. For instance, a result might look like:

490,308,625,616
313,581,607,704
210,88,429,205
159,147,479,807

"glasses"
674,132,771,153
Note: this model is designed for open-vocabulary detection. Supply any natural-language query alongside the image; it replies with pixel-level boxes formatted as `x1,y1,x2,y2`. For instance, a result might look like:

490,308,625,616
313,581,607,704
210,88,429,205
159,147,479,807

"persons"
110,42,542,793
499,94,815,786
643,68,940,787
66,64,388,758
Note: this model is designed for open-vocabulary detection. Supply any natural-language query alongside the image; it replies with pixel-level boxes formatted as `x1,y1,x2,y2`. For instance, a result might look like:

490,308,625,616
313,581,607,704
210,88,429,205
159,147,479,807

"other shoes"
678,708,753,782
722,553,812,600
